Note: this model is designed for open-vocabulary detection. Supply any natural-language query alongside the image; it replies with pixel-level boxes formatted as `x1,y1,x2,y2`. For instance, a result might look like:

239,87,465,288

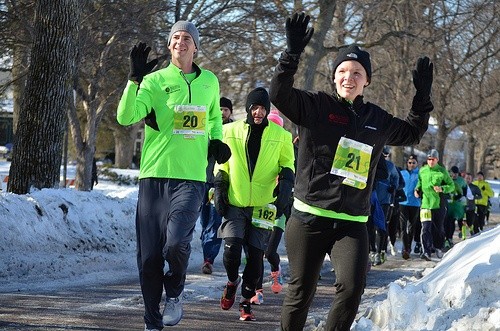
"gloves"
273,178,293,211
210,139,232,164
413,56,434,97
214,181,229,217
127,42,158,83
284,12,314,55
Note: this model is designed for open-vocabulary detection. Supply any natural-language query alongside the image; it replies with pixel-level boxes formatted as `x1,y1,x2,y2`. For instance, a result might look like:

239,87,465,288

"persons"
197,86,299,321
271,14,434,331
293,136,494,281
118,21,299,331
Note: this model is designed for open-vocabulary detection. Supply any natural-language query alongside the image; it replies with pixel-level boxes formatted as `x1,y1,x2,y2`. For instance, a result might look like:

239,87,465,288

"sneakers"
220,275,241,310
201,258,213,275
270,263,283,294
238,301,256,321
368,231,463,270
250,288,264,305
161,288,185,325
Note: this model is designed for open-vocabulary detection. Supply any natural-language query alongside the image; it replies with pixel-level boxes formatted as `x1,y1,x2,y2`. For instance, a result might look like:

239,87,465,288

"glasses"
429,159,435,161
408,162,417,164
384,155,390,157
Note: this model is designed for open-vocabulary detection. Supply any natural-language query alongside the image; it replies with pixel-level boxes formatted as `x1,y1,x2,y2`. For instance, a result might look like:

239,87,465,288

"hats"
220,97,232,113
427,149,439,160
167,21,200,52
408,154,418,161
332,46,372,87
246,87,270,115
